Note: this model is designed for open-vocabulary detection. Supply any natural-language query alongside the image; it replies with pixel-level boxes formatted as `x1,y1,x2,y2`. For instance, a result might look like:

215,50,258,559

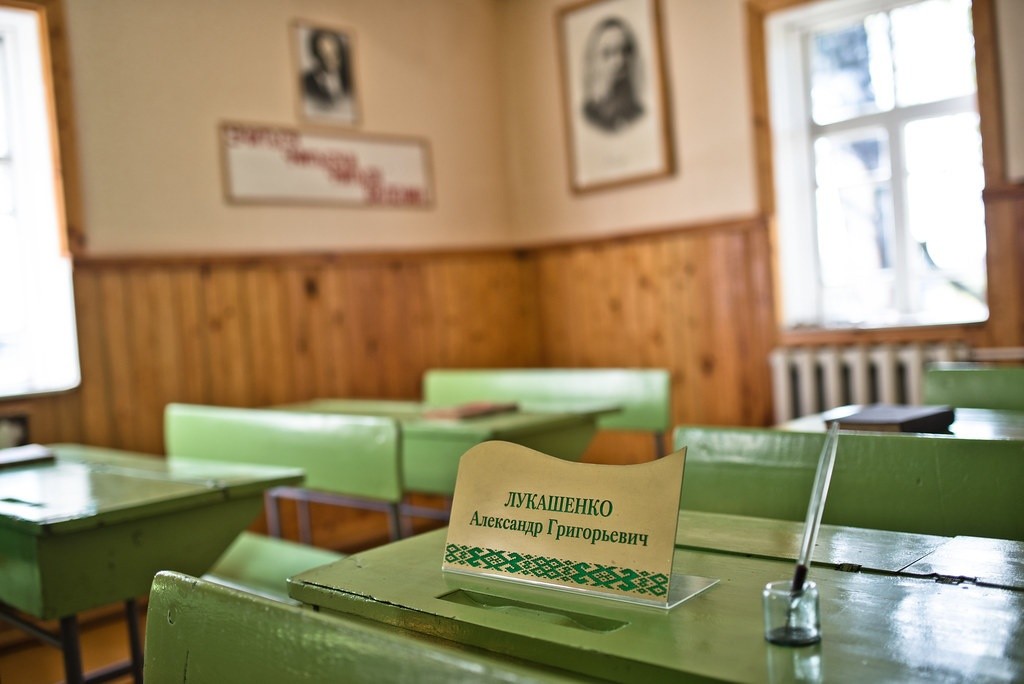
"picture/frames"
218,122,438,204
290,17,363,127
552,0,676,194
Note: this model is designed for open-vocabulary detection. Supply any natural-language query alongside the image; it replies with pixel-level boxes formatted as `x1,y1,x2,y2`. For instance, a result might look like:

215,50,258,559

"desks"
0,441,308,684
285,510,1024,684
270,398,626,525
769,404,1023,440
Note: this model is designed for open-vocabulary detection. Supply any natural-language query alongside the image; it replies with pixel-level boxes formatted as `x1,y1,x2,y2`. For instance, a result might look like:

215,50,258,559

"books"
0,443,55,467
423,400,517,423
823,405,955,434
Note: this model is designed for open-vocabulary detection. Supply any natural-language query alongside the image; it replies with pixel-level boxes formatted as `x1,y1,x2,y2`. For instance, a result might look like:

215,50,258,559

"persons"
579,17,646,135
301,30,351,110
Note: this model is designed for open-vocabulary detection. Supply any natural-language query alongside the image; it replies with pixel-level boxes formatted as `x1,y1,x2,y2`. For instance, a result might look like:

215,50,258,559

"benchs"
421,365,682,460
923,362,1023,411
664,422,1023,542
162,402,408,609
138,571,581,683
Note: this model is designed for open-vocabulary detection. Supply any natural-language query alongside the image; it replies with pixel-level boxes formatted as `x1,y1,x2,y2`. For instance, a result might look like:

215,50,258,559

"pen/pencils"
785,421,843,634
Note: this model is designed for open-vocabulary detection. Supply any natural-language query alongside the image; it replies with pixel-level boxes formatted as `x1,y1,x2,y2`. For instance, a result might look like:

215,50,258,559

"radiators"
766,339,967,427
